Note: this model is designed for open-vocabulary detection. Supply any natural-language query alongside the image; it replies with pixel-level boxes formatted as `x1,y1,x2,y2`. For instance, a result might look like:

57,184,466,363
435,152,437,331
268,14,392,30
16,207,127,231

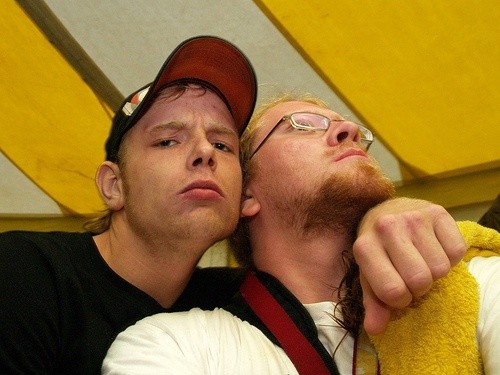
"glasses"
239,111,376,168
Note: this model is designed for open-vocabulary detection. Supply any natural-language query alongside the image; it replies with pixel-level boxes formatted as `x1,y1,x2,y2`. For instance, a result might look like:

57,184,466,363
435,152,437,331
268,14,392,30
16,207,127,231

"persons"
100,81,500,375
1,34,469,375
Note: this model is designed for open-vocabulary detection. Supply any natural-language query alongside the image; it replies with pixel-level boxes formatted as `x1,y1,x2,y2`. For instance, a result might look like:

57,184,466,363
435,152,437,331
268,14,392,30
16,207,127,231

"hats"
104,35,258,157
